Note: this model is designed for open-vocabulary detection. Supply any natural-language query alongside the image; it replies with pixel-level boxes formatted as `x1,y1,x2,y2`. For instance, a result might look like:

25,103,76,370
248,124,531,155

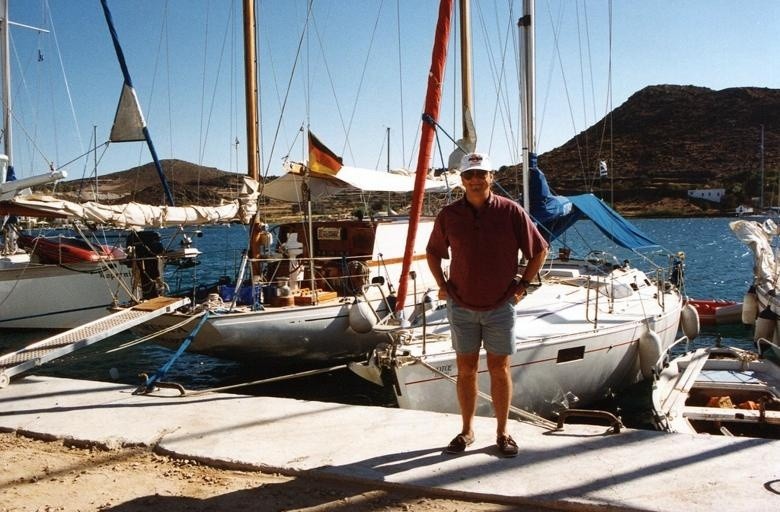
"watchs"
520,277,530,289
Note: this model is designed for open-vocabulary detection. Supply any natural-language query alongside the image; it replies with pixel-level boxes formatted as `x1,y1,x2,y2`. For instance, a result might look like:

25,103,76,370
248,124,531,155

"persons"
426,152,550,458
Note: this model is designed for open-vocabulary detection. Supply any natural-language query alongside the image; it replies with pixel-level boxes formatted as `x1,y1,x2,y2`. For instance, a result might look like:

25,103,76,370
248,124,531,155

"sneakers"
445,430,476,453
494,434,519,458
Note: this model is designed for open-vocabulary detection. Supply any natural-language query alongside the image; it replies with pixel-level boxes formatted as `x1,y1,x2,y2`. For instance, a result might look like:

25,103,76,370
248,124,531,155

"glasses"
462,171,487,180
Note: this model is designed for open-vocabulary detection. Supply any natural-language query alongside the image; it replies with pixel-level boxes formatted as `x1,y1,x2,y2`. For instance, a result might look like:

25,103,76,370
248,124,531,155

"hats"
459,152,492,173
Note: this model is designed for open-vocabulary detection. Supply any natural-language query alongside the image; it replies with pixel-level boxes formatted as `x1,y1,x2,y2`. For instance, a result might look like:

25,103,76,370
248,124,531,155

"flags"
308,127,345,177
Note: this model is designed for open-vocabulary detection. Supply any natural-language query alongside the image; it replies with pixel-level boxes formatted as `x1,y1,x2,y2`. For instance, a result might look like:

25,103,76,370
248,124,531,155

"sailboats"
346,0,684,420
107,1,452,358
0,0,203,329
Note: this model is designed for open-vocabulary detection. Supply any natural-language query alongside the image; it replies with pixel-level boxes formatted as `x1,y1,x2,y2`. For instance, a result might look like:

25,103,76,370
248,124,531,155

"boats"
682,298,743,326
649,334,780,440
